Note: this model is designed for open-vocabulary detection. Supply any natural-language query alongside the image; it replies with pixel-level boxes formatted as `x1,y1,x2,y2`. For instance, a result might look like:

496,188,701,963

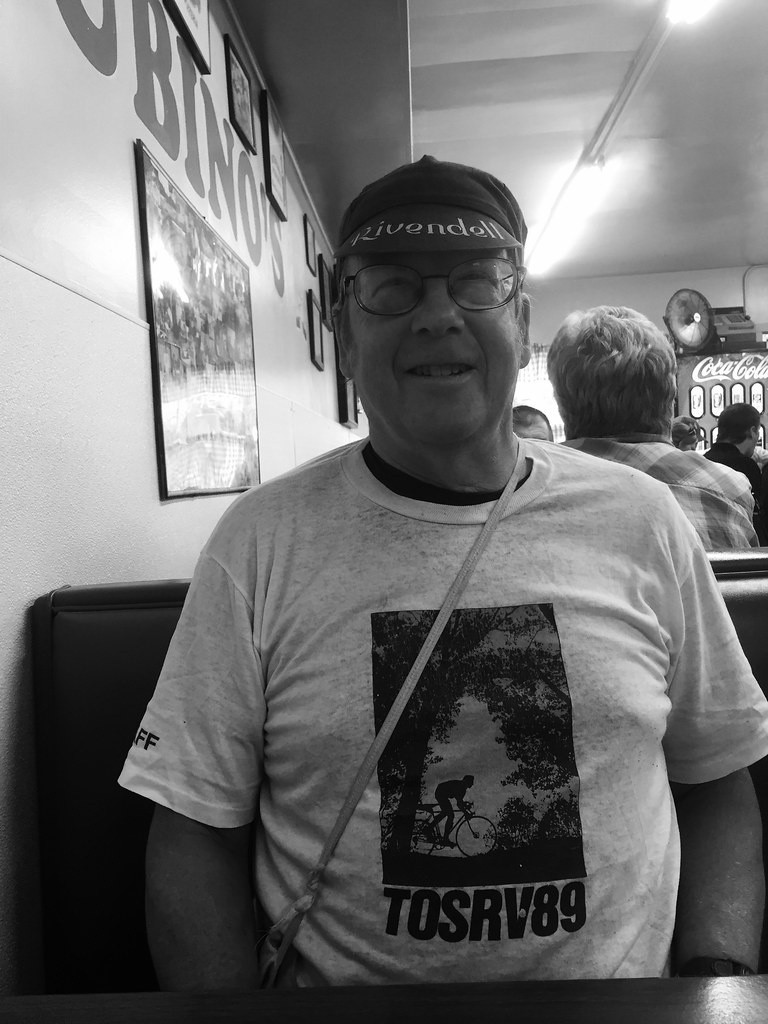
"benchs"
33,562,767,973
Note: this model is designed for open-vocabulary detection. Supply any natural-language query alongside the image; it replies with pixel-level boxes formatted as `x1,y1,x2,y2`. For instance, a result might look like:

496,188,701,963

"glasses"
335,253,526,318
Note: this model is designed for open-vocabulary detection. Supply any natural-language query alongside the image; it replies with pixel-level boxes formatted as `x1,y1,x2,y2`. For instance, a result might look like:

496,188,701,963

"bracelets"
679,956,755,978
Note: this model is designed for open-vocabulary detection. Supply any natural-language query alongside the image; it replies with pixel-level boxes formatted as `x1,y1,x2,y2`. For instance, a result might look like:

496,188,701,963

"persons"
118,157,768,995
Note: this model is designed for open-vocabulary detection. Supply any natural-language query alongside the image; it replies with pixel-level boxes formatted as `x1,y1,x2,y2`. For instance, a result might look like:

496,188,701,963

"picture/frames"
134,137,260,501
162,0,211,77
335,333,359,429
309,289,325,371
261,89,289,222
305,212,317,276
223,33,258,155
319,253,335,332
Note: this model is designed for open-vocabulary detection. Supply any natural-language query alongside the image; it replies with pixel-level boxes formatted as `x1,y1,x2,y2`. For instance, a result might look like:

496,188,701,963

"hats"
335,152,526,261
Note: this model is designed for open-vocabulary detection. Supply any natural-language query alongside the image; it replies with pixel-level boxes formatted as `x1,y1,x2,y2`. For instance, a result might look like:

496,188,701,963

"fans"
662,288,716,356
